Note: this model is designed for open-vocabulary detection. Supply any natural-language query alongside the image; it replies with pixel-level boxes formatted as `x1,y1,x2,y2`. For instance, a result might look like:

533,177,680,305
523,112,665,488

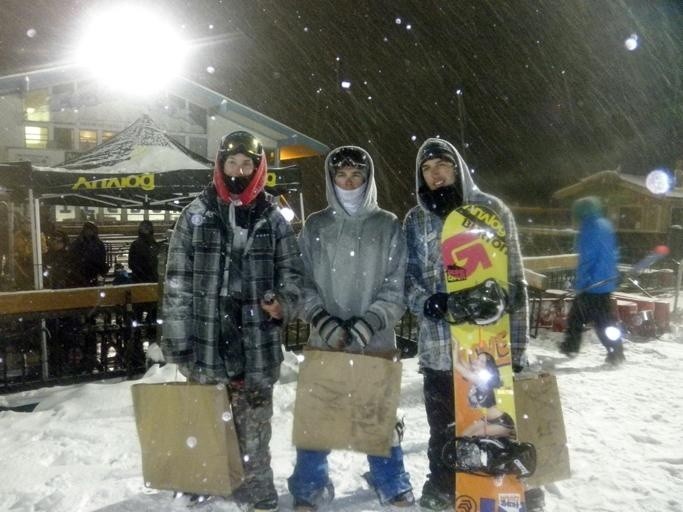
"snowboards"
441,203,528,512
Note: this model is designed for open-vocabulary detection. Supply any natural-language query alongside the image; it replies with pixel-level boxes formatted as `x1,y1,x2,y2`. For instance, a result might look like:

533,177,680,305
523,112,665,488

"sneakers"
248,479,279,512
389,489,415,507
418,481,455,512
523,488,545,510
291,496,318,512
599,352,625,370
555,341,579,358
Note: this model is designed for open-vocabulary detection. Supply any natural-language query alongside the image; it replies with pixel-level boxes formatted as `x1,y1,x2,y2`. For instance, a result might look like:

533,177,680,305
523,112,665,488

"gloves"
423,293,448,320
309,307,351,352
347,310,385,349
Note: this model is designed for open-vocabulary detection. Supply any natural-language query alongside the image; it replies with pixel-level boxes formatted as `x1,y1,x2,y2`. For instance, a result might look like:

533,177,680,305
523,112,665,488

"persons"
0,215,177,385
283,142,415,511
402,135,546,511
554,194,628,371
160,132,306,512
450,334,515,442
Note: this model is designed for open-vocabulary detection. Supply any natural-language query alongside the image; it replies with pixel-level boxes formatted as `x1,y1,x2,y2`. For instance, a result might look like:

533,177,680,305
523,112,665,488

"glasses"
220,130,264,157
328,147,369,168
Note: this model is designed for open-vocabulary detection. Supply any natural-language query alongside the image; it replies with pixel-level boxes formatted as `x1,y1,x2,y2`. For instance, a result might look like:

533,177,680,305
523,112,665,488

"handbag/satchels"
291,346,402,460
130,365,245,497
511,372,571,487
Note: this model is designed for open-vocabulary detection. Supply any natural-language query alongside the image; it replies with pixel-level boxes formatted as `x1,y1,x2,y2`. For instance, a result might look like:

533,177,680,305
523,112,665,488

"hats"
218,132,263,194
419,138,456,169
